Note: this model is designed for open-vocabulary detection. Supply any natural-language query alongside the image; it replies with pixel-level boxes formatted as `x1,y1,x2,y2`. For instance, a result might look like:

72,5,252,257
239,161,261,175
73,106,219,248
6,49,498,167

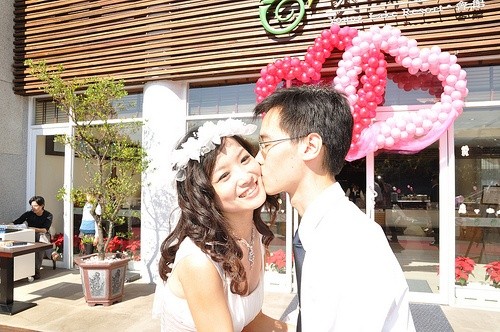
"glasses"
257,132,325,153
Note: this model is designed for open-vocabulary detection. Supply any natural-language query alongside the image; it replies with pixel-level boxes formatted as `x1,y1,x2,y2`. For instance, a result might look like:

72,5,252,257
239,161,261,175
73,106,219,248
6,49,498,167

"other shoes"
32,273,40,280
429,242,438,246
52,254,60,261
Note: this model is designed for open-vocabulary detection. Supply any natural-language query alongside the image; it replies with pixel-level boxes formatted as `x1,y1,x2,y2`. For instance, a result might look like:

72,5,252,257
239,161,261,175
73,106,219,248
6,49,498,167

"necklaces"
231,223,257,270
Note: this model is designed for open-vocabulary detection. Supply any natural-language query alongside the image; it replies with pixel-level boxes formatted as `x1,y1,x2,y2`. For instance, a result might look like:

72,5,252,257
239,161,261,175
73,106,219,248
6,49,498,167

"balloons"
255,24,469,156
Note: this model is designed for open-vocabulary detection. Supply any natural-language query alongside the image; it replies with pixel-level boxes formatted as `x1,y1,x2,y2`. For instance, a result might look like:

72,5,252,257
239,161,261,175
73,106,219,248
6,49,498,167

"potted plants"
26,59,148,305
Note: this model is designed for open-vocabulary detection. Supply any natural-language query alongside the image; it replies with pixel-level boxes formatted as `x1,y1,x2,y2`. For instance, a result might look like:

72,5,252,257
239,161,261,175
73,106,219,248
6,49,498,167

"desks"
0,242,53,315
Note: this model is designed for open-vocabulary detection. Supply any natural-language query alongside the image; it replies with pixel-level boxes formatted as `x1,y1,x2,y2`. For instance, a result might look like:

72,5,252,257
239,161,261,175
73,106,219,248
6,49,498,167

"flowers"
265,249,288,273
168,117,256,181
51,233,141,261
484,261,500,289
456,255,476,287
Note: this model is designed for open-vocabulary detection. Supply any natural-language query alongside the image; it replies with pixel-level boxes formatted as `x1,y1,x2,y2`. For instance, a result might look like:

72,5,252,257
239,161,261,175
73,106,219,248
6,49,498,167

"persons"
251,81,415,332
157,117,288,332
344,168,439,246
79,188,102,261
2,195,61,280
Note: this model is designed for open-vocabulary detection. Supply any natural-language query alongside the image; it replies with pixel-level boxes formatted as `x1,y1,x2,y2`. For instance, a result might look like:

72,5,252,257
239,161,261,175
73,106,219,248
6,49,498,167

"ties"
292,229,306,332
